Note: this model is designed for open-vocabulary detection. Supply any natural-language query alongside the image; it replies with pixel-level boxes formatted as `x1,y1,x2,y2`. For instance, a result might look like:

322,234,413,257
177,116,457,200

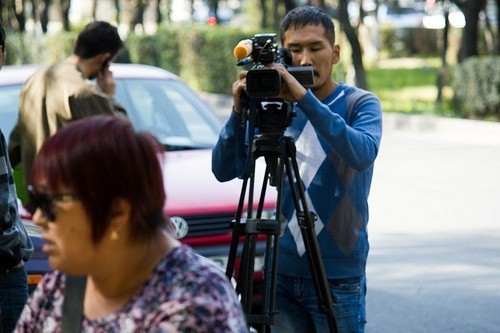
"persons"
212,6,382,333
8,20,127,195
0,129,35,333
12,117,244,333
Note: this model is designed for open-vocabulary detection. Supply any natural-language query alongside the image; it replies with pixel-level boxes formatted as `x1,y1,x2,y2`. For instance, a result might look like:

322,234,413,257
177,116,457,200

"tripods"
225,97,339,333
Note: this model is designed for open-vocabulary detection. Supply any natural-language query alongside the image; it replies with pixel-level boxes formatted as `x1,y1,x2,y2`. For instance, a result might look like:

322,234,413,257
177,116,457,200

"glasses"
25,185,79,221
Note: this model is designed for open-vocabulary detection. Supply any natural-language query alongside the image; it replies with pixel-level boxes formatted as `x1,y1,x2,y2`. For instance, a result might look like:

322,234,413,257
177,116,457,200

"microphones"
234,39,253,59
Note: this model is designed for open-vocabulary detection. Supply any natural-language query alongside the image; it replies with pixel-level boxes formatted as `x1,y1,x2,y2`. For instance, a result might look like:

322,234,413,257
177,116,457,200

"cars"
0,63,278,291
379,5,465,28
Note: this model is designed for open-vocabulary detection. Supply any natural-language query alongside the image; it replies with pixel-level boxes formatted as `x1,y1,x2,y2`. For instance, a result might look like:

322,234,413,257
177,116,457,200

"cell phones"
102,58,112,72
